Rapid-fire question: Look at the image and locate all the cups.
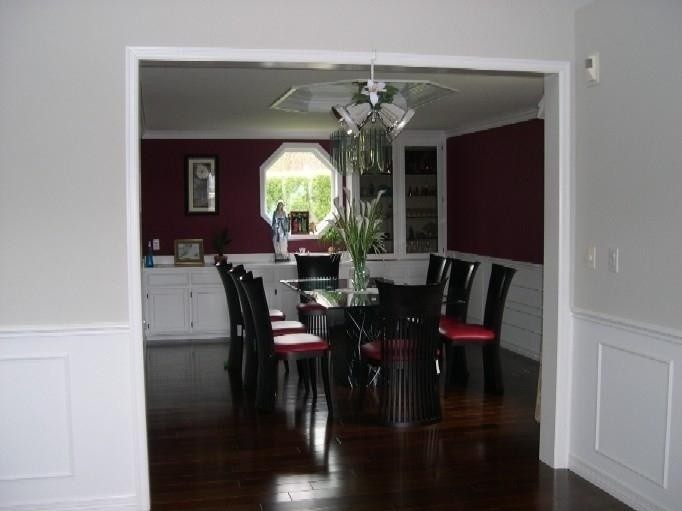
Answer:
[407,207,434,217]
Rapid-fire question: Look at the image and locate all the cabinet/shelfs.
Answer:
[142,264,190,340]
[335,129,450,261]
[191,262,230,340]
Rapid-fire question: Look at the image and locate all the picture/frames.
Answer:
[171,239,206,266]
[186,156,219,214]
[290,210,309,235]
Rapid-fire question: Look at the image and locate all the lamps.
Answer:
[329,48,416,174]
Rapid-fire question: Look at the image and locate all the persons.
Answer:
[291,217,307,232]
[271,202,290,262]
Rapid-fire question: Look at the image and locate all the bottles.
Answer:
[146,241,154,266]
[408,184,436,197]
[407,227,432,252]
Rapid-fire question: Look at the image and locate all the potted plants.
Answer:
[213,224,232,265]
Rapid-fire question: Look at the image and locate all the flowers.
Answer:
[325,189,392,292]
[351,79,400,112]
